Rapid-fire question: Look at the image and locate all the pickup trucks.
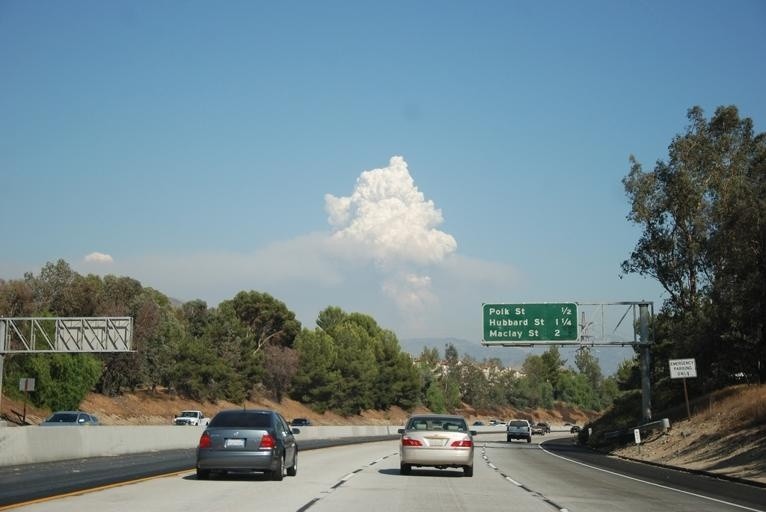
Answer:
[175,410,209,426]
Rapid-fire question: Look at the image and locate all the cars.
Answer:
[195,409,300,481]
[397,414,473,476]
[531,423,550,435]
[571,426,580,433]
[292,418,308,426]
[507,419,531,442]
[474,419,496,425]
[39,411,98,425]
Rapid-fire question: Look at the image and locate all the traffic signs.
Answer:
[482,301,581,344]
